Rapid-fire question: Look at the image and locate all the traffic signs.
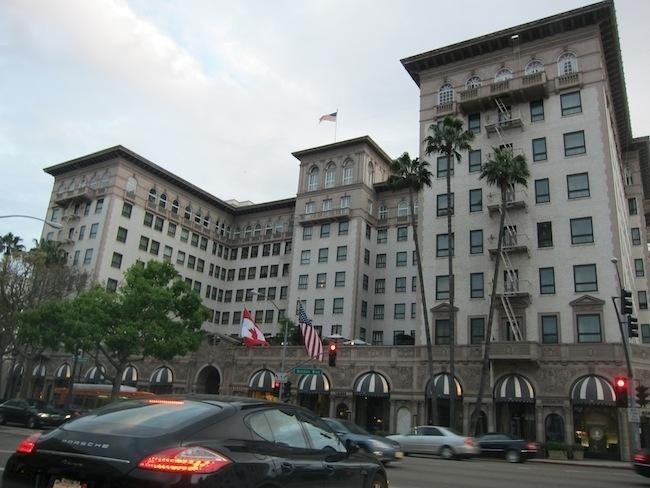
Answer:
[294,369,321,374]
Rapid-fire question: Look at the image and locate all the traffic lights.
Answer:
[274,381,280,390]
[615,377,629,409]
[621,288,633,316]
[635,386,649,407]
[328,343,337,367]
[629,318,638,337]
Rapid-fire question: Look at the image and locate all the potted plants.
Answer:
[543,441,587,461]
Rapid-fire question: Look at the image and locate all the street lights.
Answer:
[1,214,62,230]
[250,288,289,402]
[610,258,633,378]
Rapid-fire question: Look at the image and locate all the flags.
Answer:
[319,112,336,122]
[241,307,271,347]
[298,303,324,362]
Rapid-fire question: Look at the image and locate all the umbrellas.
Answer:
[324,331,347,340]
[343,336,372,346]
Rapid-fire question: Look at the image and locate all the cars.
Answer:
[475,432,539,463]
[387,425,482,461]
